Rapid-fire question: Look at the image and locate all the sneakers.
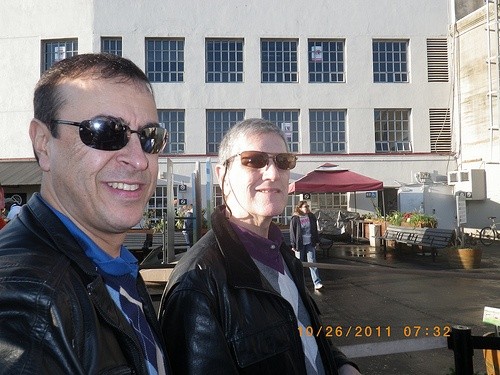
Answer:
[314,283,323,289]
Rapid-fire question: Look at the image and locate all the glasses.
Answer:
[0,208,6,214]
[222,151,298,170]
[301,205,310,208]
[53,115,169,154]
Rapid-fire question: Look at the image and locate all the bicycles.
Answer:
[480,217,500,246]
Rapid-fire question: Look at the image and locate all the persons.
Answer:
[156,118,363,374]
[0,52,167,375]
[0,185,23,230]
[179,204,195,252]
[290,200,325,290]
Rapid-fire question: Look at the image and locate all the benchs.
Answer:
[379,225,454,262]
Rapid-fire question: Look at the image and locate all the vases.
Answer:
[458,248,482,269]
